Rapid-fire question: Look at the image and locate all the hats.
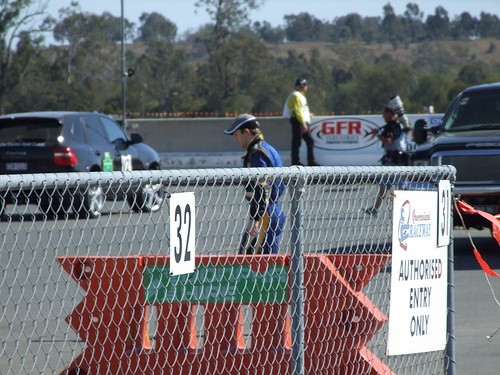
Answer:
[224,114,260,135]
[296,79,308,88]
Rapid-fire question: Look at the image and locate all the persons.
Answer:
[224,113,286,255]
[283,77,322,166]
[363,107,408,218]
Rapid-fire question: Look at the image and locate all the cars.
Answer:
[407,80,500,230]
[1,111,164,220]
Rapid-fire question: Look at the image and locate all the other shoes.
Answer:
[363,206,378,218]
[309,162,321,166]
[291,162,304,166]
